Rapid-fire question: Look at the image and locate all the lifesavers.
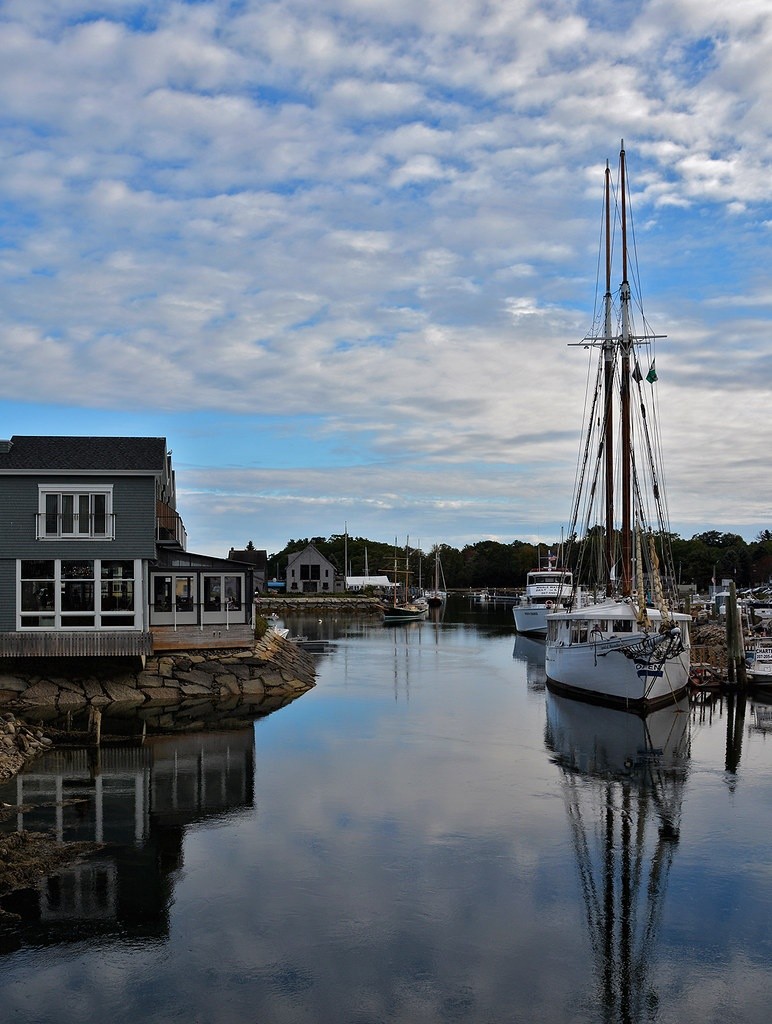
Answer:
[546,600,553,606]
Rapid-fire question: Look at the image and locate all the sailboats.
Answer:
[512,137,772,717]
[381,532,446,625]
